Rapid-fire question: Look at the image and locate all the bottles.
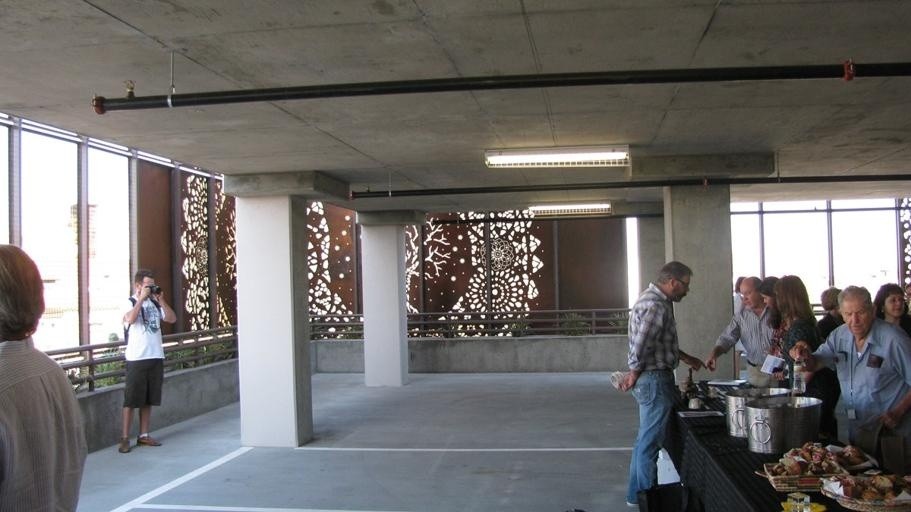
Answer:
[793,355,807,393]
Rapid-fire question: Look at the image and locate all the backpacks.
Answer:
[123,296,145,345]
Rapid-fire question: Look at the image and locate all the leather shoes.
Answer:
[137,434,162,447]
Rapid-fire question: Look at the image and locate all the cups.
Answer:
[689,398,701,409]
[611,372,625,389]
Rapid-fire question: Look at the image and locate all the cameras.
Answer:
[144,285,161,295]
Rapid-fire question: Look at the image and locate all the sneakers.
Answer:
[626,501,639,509]
[119,437,130,453]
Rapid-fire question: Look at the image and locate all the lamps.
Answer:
[527,198,612,217]
[484,143,632,168]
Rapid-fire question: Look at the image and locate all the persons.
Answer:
[705,274,911,476]
[0,243,89,512]
[118,269,177,452]
[618,260,707,506]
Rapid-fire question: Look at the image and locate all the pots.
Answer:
[724,387,790,438]
[743,396,824,455]
